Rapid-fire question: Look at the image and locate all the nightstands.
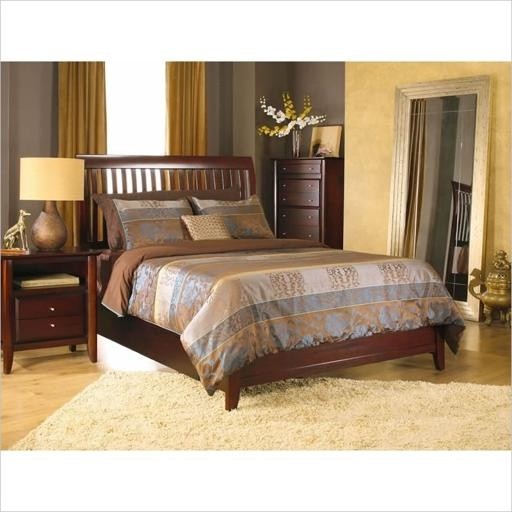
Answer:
[0,247,104,375]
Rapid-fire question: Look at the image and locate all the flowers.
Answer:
[256,88,328,138]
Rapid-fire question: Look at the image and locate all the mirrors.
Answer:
[384,71,497,325]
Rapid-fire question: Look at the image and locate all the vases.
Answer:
[291,128,305,158]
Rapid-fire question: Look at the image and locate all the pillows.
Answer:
[109,194,194,251]
[91,185,243,252]
[180,209,235,241]
[185,190,277,240]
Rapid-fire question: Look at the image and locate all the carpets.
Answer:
[8,365,512,451]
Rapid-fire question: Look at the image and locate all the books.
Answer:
[14,272,81,290]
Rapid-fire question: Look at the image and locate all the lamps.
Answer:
[17,156,87,253]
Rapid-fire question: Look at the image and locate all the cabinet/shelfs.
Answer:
[270,155,345,250]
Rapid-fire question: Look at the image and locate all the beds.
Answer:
[72,153,473,416]
[442,178,472,301]
